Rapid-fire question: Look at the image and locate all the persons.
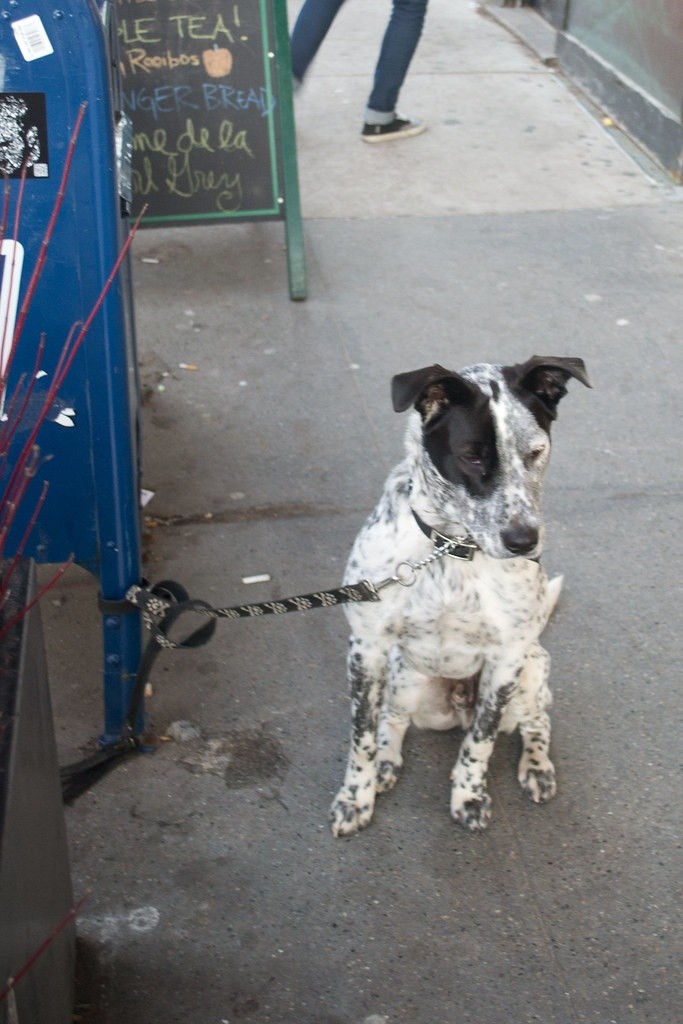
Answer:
[291,0,429,143]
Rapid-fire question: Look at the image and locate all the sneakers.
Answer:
[359,113,425,142]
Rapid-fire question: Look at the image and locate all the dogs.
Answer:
[327,353,594,839]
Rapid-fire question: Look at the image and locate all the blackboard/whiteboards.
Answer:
[107,0,286,221]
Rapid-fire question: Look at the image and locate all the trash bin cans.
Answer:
[0,0,184,794]
